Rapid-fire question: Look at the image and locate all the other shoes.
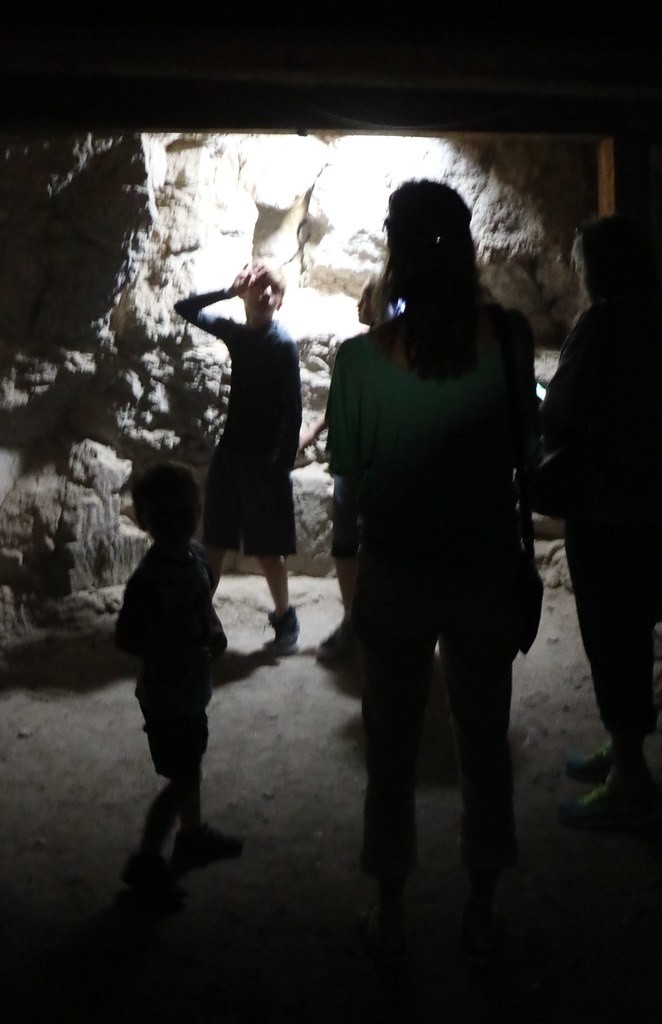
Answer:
[464,908,507,963]
[359,910,407,961]
[121,855,189,903]
[316,628,354,663]
[172,822,244,861]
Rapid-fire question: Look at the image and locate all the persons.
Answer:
[106,456,242,898]
[176,270,302,647]
[296,180,662,988]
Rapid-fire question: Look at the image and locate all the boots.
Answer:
[266,606,300,653]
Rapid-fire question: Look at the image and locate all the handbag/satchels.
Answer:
[516,549,544,655]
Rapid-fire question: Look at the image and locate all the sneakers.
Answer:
[565,743,613,780]
[560,768,658,827]
